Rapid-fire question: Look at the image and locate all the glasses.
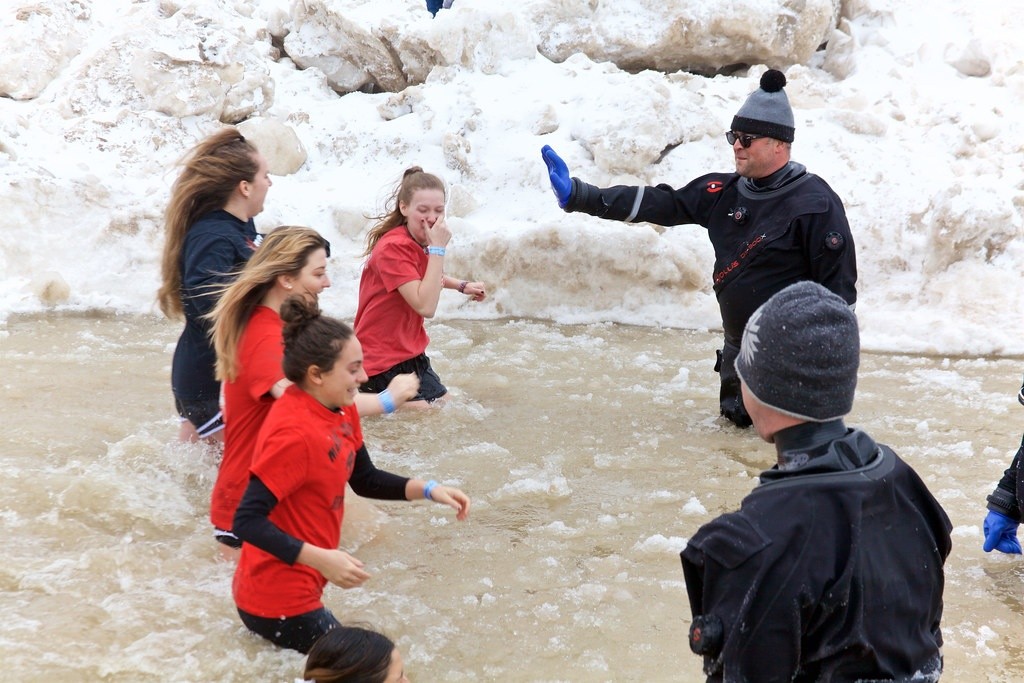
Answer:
[726,130,769,148]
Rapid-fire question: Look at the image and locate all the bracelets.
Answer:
[427,246,446,257]
[422,478,439,501]
[458,281,469,292]
[377,388,396,413]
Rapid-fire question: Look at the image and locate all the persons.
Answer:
[302,626,412,683]
[160,124,274,453]
[349,160,487,412]
[674,280,956,682]
[229,292,472,667]
[979,384,1024,553]
[187,225,334,561]
[542,65,861,437]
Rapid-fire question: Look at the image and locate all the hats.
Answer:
[731,69,795,143]
[734,279,861,420]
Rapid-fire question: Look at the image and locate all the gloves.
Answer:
[542,144,572,207]
[983,510,1022,555]
